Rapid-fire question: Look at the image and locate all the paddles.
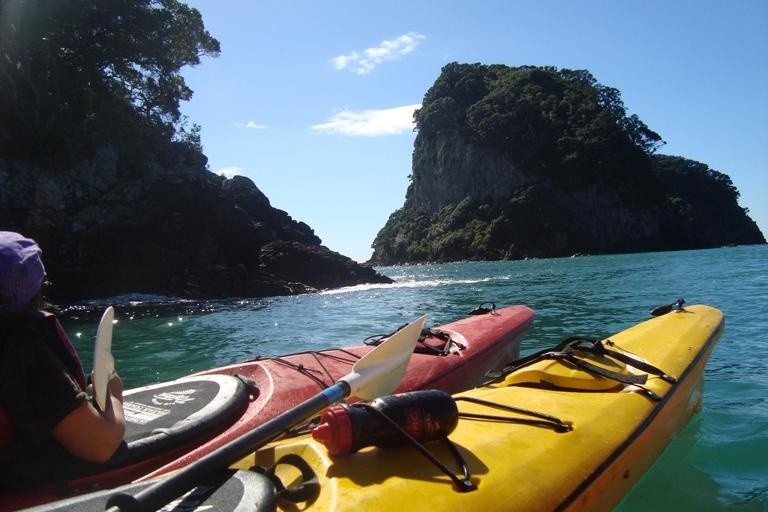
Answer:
[103,312,428,512]
[92,304,115,412]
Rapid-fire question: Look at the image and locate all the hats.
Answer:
[0,230,46,310]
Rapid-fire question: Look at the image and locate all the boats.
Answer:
[3,299,540,510]
[8,297,732,510]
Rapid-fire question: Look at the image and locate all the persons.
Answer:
[2,229,127,498]
